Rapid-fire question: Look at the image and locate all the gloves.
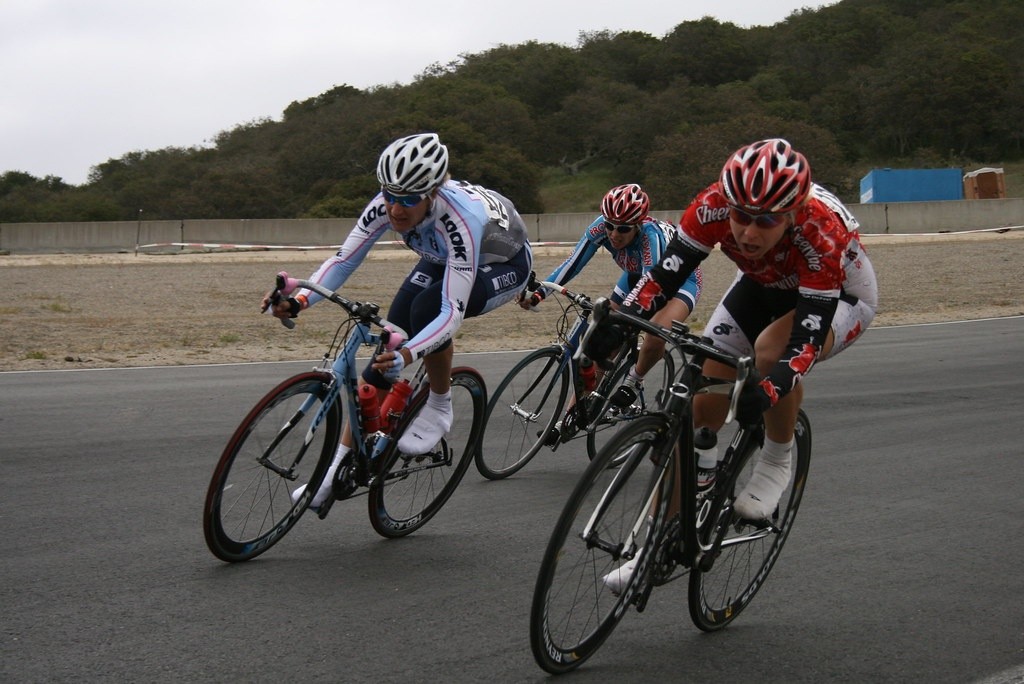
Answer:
[730,372,787,414]
[586,323,628,360]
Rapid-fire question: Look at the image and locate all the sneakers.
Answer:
[610,376,644,407]
[537,425,563,447]
[398,406,453,452]
[603,546,644,595]
[733,460,791,518]
[292,468,332,510]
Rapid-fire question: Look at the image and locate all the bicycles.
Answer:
[202,270,490,564]
[473,270,677,482]
[528,294,814,679]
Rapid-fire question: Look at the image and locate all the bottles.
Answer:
[380,379,414,434]
[579,356,598,392]
[359,384,381,432]
[695,425,718,493]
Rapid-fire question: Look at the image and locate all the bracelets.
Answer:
[534,293,543,303]
[299,295,309,309]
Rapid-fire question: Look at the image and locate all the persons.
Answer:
[263,131,532,510]
[514,182,701,446]
[600,139,879,596]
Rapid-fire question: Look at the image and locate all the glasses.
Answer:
[380,188,428,205]
[604,219,635,235]
[730,206,786,230]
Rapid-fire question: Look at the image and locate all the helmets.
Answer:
[721,137,810,211]
[376,133,448,194]
[602,183,650,224]
